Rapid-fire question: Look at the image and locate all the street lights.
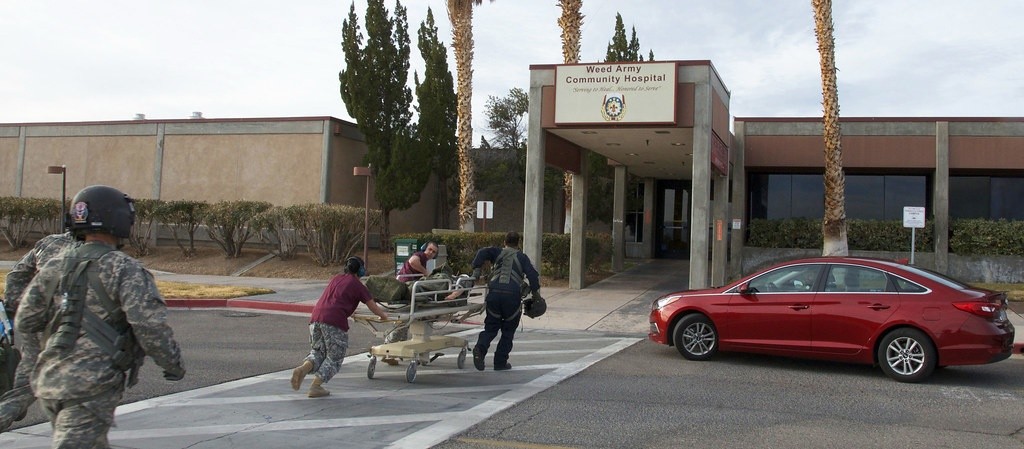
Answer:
[45,165,67,233]
[352,162,372,278]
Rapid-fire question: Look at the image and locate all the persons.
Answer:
[469,231,541,371]
[0,184,188,449]
[290,256,387,397]
[382,240,439,366]
[402,270,475,307]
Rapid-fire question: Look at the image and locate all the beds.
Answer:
[350,264,490,384]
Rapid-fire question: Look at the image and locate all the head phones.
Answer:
[343,257,365,277]
[421,240,439,259]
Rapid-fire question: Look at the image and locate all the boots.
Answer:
[291,361,313,391]
[307,374,330,398]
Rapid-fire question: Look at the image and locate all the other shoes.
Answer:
[474,348,485,371]
[493,363,511,370]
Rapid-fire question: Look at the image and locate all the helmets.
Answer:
[70,185,136,239]
[524,297,547,318]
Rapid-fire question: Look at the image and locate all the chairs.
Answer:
[843,272,860,292]
[824,272,840,292]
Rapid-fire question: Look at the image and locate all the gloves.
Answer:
[162,360,184,382]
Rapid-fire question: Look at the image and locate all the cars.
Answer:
[647,255,1017,385]
